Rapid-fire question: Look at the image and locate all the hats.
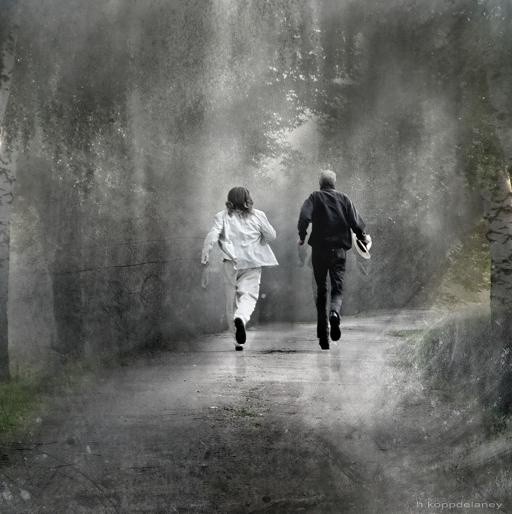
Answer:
[352,233,372,260]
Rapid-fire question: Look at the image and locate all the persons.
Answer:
[296,169,368,349]
[199,184,278,351]
[297,226,330,310]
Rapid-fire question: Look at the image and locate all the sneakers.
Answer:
[330,311,341,341]
[234,318,246,345]
